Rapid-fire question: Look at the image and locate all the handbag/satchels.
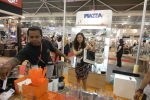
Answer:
[83,49,96,64]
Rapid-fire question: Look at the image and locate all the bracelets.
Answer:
[136,88,143,93]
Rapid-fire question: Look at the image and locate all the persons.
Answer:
[49,31,71,61]
[72,33,96,90]
[134,64,150,100]
[0,25,68,82]
[115,38,124,67]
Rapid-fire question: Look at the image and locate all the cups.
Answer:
[14,61,65,100]
